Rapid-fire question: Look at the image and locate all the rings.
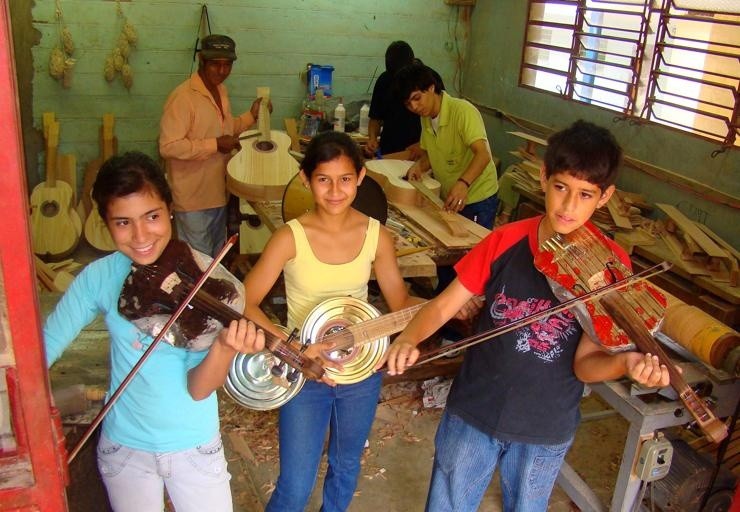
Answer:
[458,199,463,206]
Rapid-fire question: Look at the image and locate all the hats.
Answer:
[199,35,237,60]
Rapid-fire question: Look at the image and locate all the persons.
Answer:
[41,151,266,512]
[392,65,501,232]
[240,129,485,512]
[158,34,274,259]
[371,120,684,512]
[368,40,426,154]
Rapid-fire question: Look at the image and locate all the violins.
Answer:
[117,239,325,383]
[533,221,729,444]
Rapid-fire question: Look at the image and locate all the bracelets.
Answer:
[457,178,472,188]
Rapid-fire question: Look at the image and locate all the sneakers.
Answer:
[439,338,460,358]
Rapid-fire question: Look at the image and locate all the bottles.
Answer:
[333,103,346,133]
[359,101,370,137]
